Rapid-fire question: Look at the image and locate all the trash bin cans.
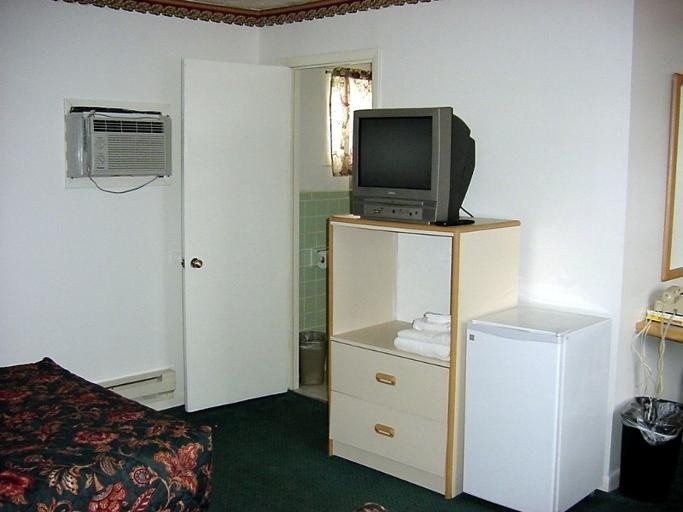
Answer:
[619,397,683,502]
[299,330,327,386]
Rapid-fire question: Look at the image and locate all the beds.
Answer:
[0,358,217,511]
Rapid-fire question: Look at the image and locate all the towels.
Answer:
[392,310,451,365]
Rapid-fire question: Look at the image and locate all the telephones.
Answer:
[654,284,683,319]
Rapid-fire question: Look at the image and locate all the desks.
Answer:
[634,319,682,344]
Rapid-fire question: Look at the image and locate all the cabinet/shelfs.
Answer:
[325,212,522,501]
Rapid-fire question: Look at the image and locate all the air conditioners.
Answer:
[63,107,174,182]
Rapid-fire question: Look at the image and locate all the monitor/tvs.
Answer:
[352,107,475,223]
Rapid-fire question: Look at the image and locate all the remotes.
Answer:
[435,219,475,226]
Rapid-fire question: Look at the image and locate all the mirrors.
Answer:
[660,72,682,282]
